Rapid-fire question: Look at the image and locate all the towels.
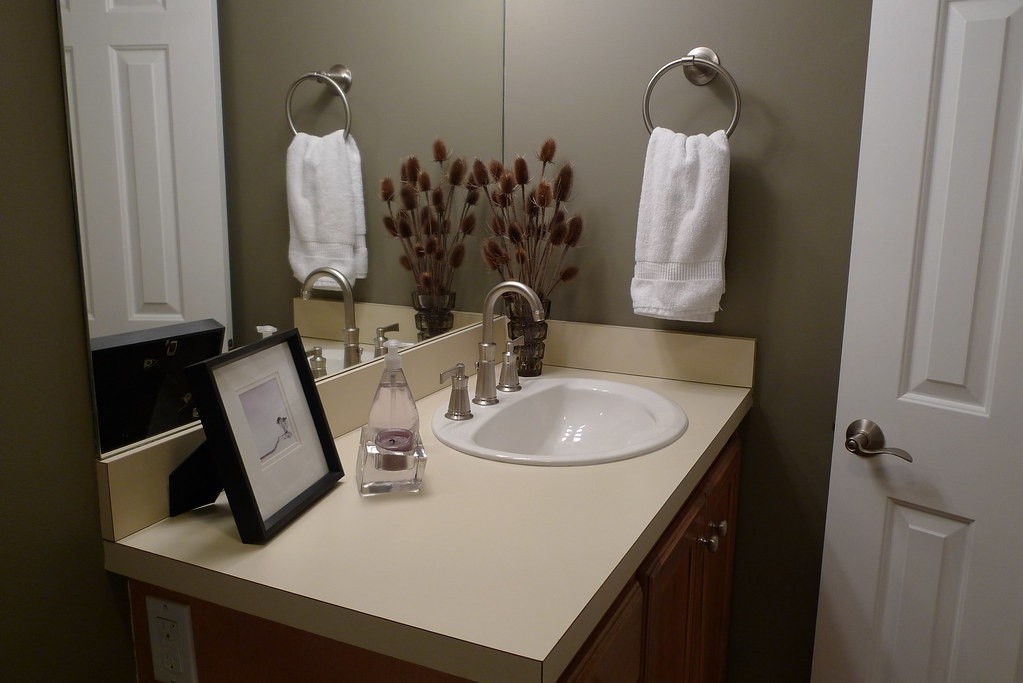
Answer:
[282,129,368,291]
[630,125,733,322]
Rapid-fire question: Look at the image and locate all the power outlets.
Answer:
[145,596,198,683]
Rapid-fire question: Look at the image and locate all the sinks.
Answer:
[430,377,691,467]
[301,346,375,375]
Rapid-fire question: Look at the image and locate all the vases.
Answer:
[411,291,457,344]
[504,300,551,378]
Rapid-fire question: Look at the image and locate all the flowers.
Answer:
[378,140,479,336]
[472,138,584,322]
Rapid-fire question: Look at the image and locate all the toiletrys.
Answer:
[254,325,278,339]
[369,337,419,430]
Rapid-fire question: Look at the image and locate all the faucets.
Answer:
[472,281,546,406]
[300,267,363,369]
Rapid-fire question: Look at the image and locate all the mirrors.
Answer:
[56,0,505,460]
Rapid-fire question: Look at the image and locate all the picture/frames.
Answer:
[184,327,344,545]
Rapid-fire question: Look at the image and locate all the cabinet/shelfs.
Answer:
[560,583,643,683]
[643,437,745,683]
